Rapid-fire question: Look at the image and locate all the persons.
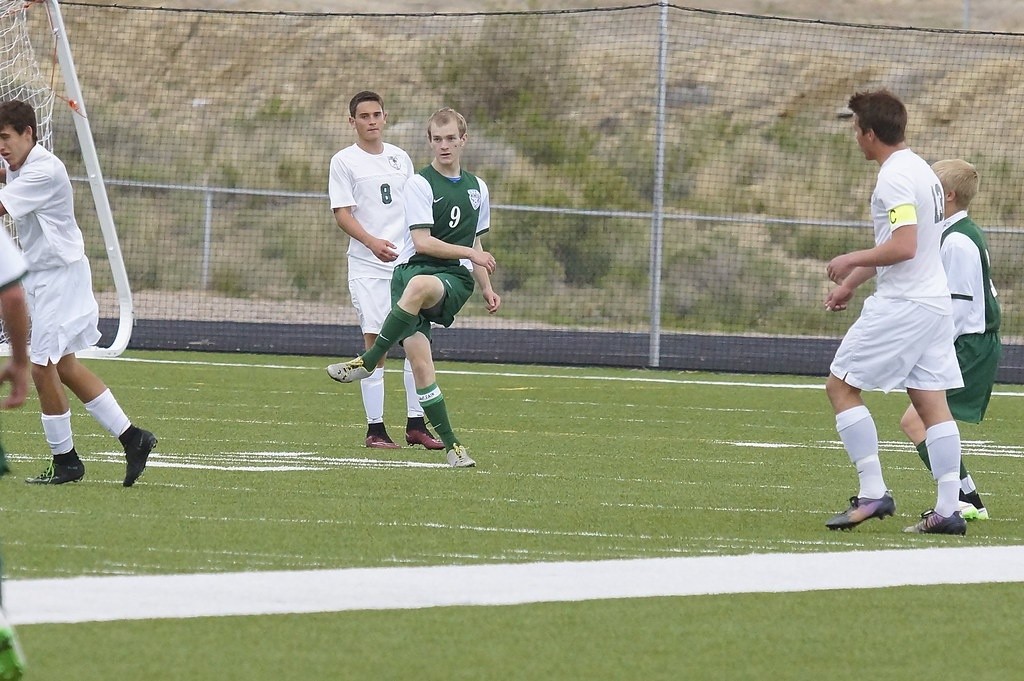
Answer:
[898,160,1001,520]
[328,90,446,451]
[0,102,158,487]
[1,226,30,407]
[824,90,969,535]
[325,106,502,468]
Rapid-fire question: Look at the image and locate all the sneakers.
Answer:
[26,448,85,485]
[825,489,897,532]
[117,425,157,487]
[921,500,989,520]
[365,423,401,448]
[406,417,445,450]
[326,355,378,383]
[445,443,477,468]
[902,507,968,537]
